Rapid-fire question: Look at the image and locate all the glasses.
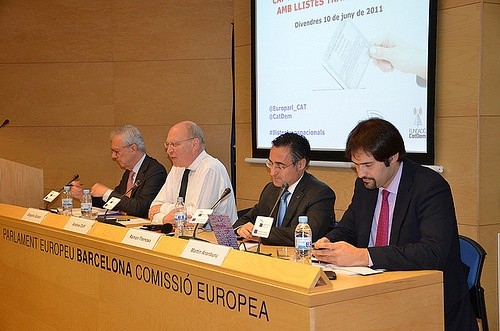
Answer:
[164,138,195,148]
[265,159,301,171]
[111,143,137,157]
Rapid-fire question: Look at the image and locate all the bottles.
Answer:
[173,197,187,236]
[79,189,92,219]
[61,186,73,216]
[295,216,312,263]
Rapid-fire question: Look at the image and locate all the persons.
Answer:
[148,120,238,231]
[309,118,479,331]
[231,133,336,246]
[69,125,167,215]
[369,37,426,79]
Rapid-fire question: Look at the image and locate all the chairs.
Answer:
[459,235,489,331]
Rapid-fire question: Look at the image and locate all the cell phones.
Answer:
[323,271,336,280]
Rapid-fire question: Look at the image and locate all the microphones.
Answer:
[178,188,231,243]
[97,185,137,227]
[39,174,79,214]
[247,182,289,255]
[0,119,10,127]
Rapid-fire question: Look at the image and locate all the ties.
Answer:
[177,169,191,206]
[375,189,390,246]
[126,172,136,198]
[276,192,291,227]
